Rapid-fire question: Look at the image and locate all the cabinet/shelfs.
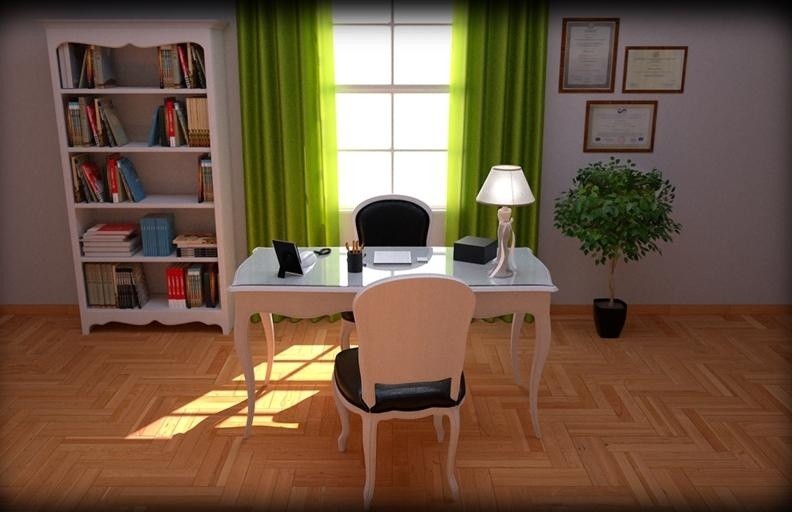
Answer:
[37,15,250,337]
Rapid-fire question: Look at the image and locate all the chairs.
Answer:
[334,274,478,511]
[340,197,432,350]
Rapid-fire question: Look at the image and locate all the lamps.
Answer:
[474,164,537,280]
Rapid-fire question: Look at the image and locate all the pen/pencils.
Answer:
[345,241,365,256]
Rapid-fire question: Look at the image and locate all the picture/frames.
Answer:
[557,16,690,154]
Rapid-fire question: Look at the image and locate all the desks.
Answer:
[226,245,559,439]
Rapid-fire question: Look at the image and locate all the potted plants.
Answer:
[552,155,683,341]
